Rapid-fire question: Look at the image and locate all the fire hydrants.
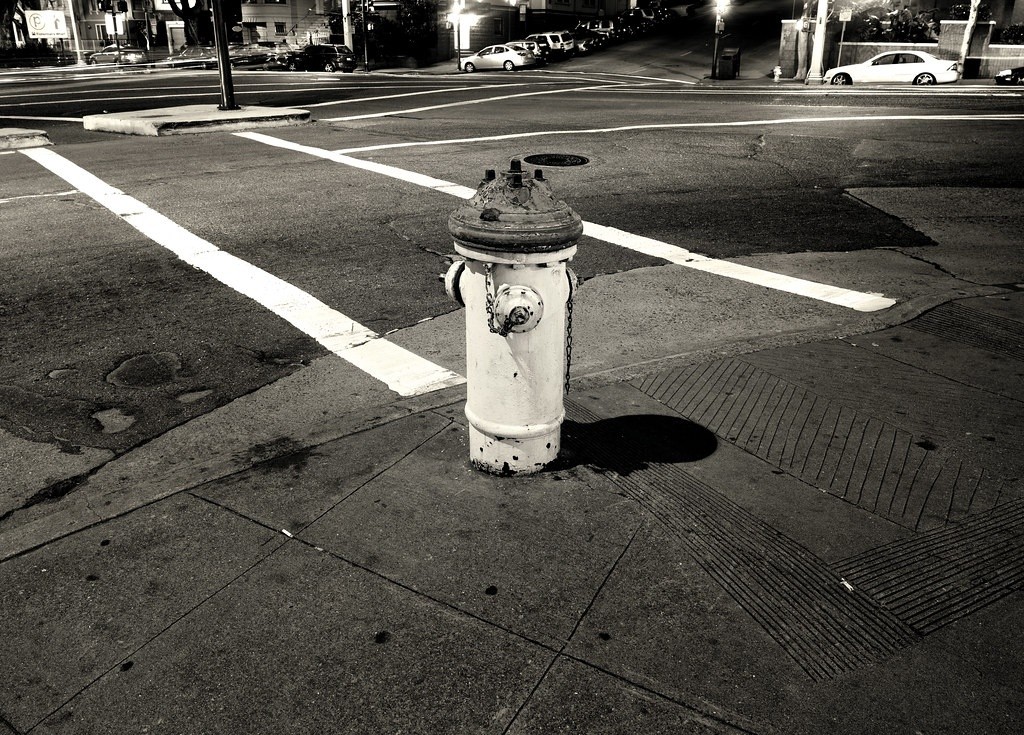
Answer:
[772,65,782,82]
[438,156,583,479]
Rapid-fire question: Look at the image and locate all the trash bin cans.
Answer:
[719,48,741,79]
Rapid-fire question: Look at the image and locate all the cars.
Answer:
[501,1,676,68]
[994,66,1024,85]
[460,43,537,73]
[88,43,149,69]
[822,48,961,86]
[166,40,359,73]
